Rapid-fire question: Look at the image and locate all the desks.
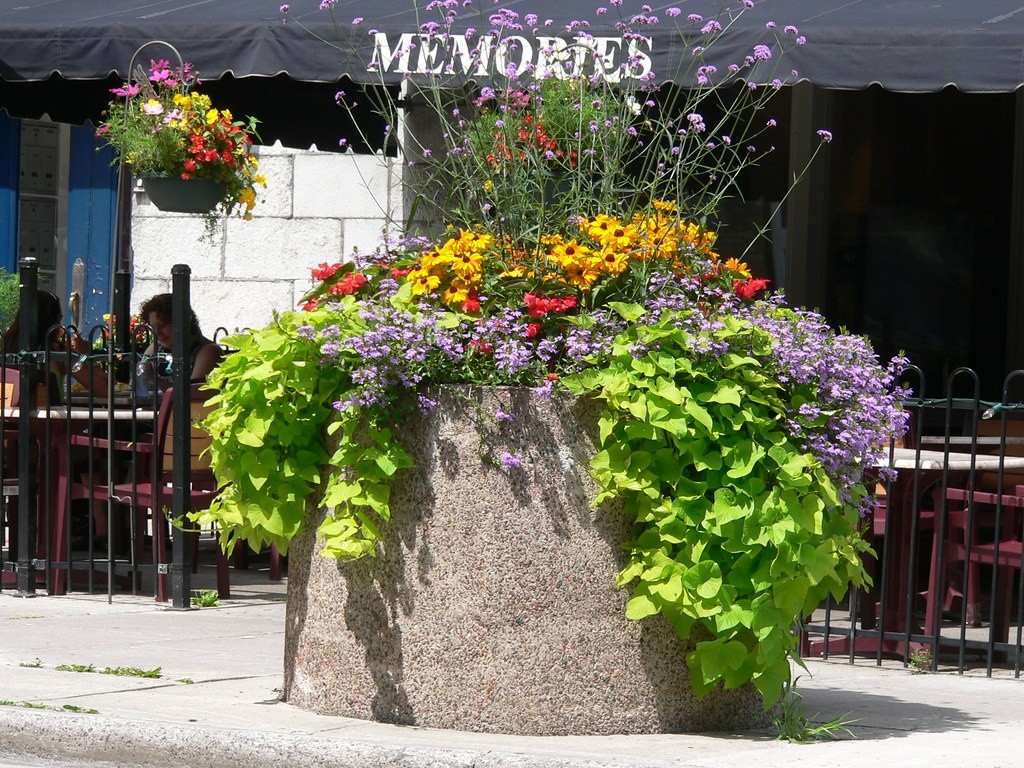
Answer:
[1,405,159,594]
[809,444,1024,670]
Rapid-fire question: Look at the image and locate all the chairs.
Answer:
[925,483,1024,663]
[0,366,20,407]
[48,381,230,601]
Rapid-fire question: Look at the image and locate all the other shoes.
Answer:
[71,533,108,553]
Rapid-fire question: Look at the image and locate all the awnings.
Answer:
[0,0,1024,158]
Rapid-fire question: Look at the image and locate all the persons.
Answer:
[0,289,109,555]
[130,294,224,395]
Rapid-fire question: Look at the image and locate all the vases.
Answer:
[140,173,227,213]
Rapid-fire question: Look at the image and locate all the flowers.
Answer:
[102,312,147,343]
[93,56,268,221]
